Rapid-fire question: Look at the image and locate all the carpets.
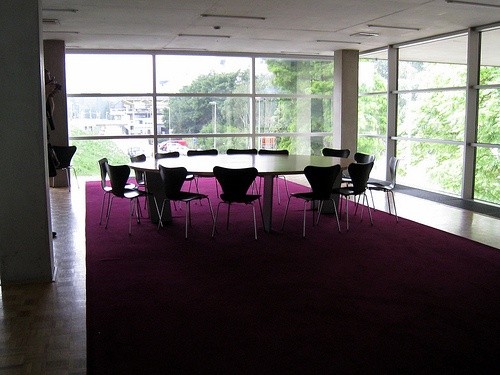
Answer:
[85,175,500,375]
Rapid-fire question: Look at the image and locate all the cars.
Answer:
[158,140,200,154]
[69,119,153,162]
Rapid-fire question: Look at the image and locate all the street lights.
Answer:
[156,106,174,159]
[209,101,217,149]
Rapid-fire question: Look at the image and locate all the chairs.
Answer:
[98,147,399,240]
[50,145,80,192]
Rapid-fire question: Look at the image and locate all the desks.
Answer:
[128,154,358,232]
